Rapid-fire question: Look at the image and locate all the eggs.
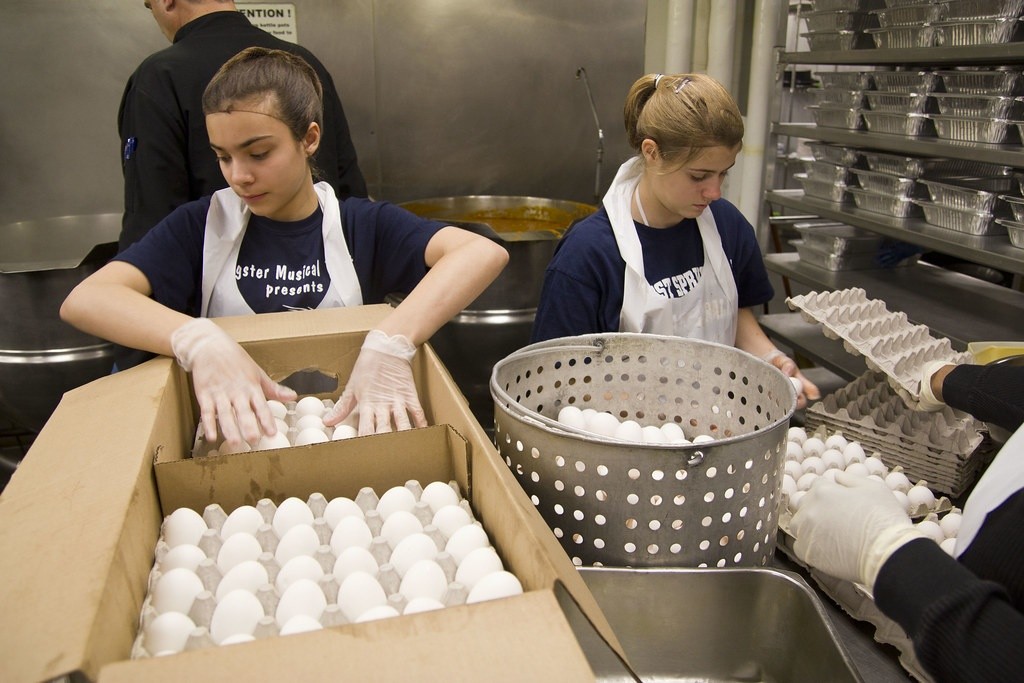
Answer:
[143,481,523,655]
[219,396,358,453]
[780,427,961,556]
[556,405,716,443]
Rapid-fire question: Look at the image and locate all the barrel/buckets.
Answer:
[384,195,600,431]
[0,215,128,487]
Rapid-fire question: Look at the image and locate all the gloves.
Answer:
[790,471,937,598]
[899,359,956,413]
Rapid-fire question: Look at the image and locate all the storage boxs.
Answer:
[0,303,641,683]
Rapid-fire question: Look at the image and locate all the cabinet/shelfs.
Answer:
[759,0,1024,418]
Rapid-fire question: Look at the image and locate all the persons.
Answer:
[532,73,823,408]
[788,352,1024,683]
[75,0,367,268]
[57,46,510,451]
